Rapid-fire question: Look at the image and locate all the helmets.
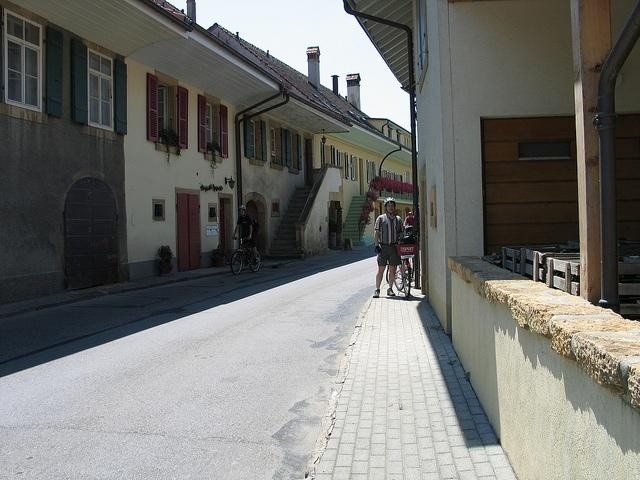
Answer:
[238,205,246,211]
[384,197,396,207]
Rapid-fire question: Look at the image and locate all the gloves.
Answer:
[374,246,381,253]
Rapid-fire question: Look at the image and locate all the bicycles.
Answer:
[386,223,419,301]
[229,236,262,275]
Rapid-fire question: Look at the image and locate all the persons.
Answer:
[403,211,415,228]
[371,196,403,298]
[232,204,259,266]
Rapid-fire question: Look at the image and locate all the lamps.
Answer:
[225,176,235,189]
[322,128,326,144]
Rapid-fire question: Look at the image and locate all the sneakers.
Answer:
[253,256,259,265]
[373,289,379,298]
[387,288,395,296]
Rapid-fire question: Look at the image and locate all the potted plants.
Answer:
[156,246,173,274]
[211,247,225,267]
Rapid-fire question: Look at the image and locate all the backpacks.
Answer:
[247,215,259,231]
